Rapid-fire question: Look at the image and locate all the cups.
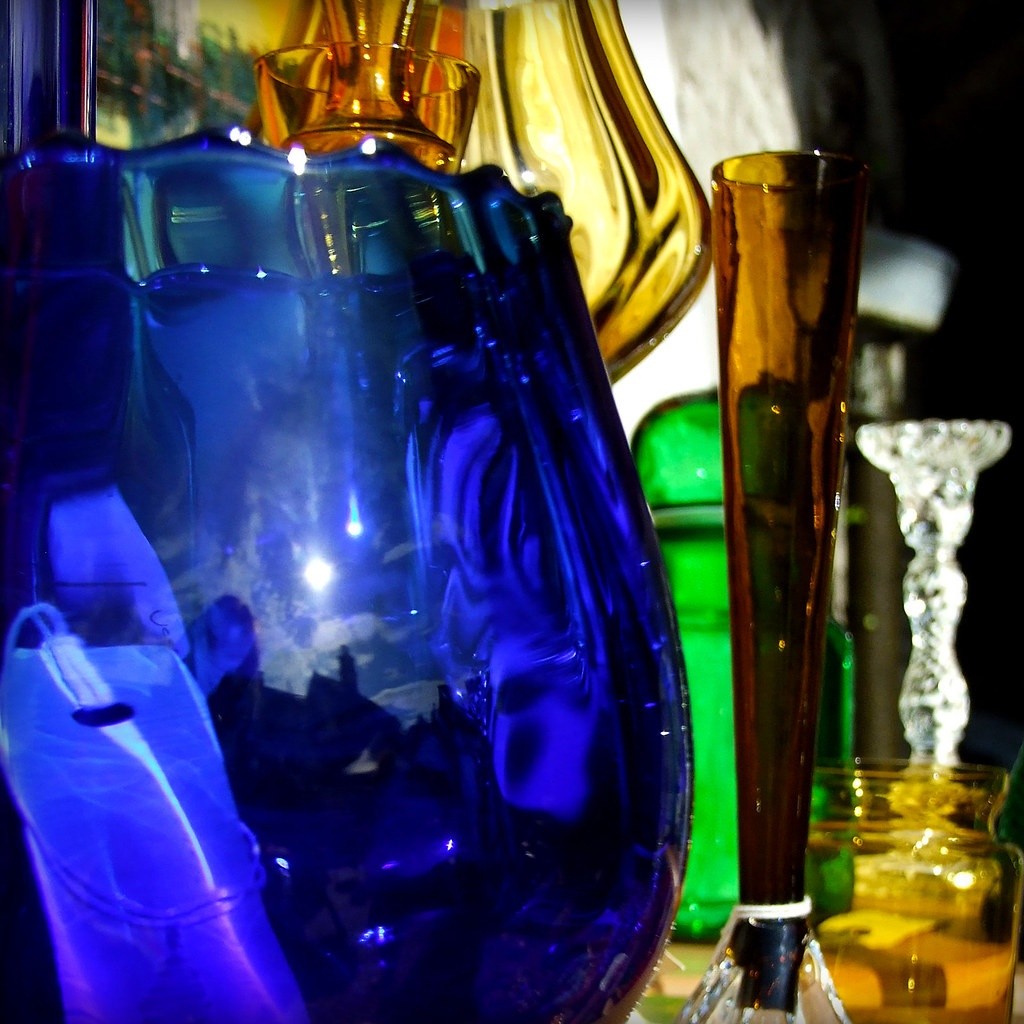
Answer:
[806,754,1024,1024]
[209,1,723,389]
[709,151,871,922]
[0,131,695,1023]
